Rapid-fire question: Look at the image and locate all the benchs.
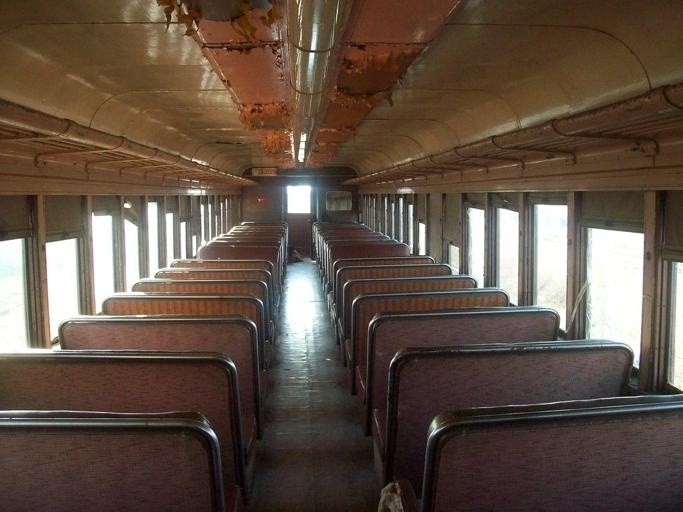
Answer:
[329,256,435,309]
[333,263,453,344]
[1,410,242,512]
[101,292,272,371]
[132,279,274,342]
[197,220,289,260]
[344,288,509,394]
[373,339,634,487]
[312,221,410,259]
[355,306,561,436]
[170,259,273,268]
[0,347,259,509]
[394,393,683,512]
[339,275,479,366]
[154,267,274,320]
[59,315,267,441]
